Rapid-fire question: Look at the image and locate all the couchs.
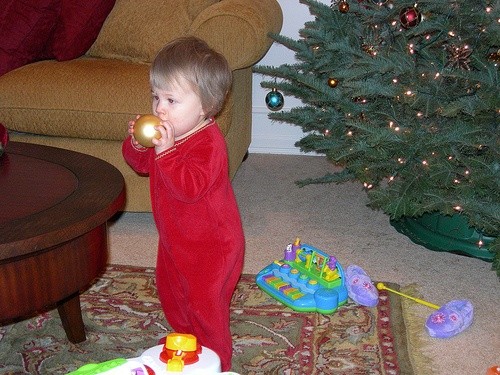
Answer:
[0,0,283,211]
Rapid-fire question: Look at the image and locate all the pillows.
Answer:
[0,0,116,77]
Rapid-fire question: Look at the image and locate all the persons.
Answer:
[120,38,246,372]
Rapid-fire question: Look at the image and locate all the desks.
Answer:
[0,142,127,343]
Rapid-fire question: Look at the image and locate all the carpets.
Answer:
[0,263,439,375]
